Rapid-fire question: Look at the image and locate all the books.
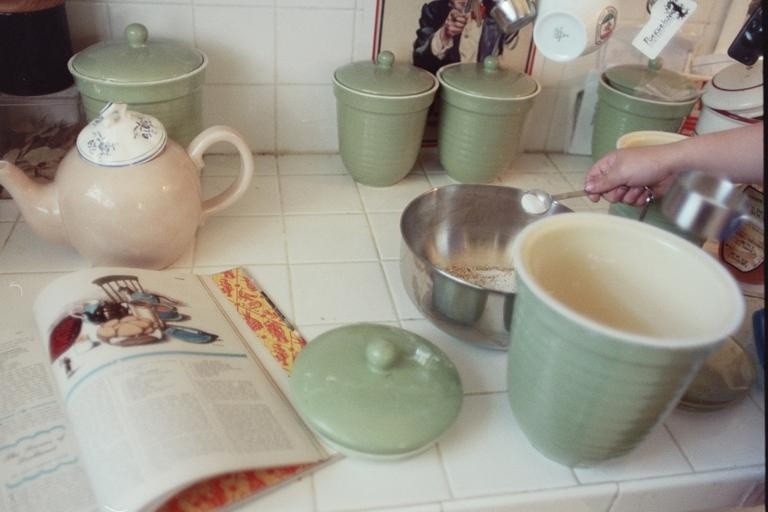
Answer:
[0,265,344,512]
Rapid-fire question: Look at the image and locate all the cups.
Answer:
[507,211,747,469]
[66,23,209,145]
[608,130,713,250]
[692,58,764,140]
[332,50,439,189]
[437,58,542,185]
[591,58,702,167]
[533,0,618,63]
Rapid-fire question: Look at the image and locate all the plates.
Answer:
[676,337,759,411]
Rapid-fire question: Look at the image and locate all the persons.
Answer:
[585,120,764,207]
[412,0,520,75]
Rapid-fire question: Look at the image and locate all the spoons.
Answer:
[520,181,656,215]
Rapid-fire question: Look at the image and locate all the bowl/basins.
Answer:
[490,0,535,35]
[400,184,573,352]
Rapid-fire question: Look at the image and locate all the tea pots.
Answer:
[83,301,129,324]
[0,102,253,271]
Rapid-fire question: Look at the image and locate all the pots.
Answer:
[661,170,764,242]
